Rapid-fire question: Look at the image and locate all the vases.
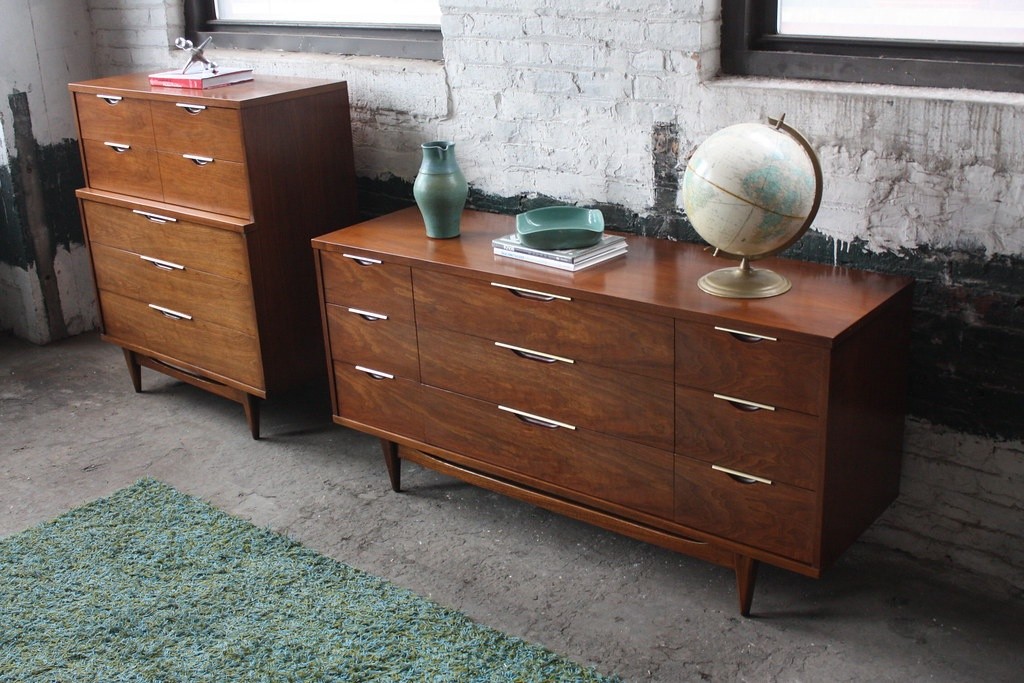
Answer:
[413,139,469,238]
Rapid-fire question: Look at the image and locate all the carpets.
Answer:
[0,476,625,683]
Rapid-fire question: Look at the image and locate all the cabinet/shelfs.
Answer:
[66,70,358,440]
[310,205,914,616]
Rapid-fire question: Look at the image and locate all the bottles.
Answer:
[413,141,468,238]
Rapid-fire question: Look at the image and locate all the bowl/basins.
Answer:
[517,206,605,250]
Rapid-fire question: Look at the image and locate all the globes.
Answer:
[681,113,824,299]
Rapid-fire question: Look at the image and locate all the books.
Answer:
[148,66,254,89]
[491,233,628,272]
